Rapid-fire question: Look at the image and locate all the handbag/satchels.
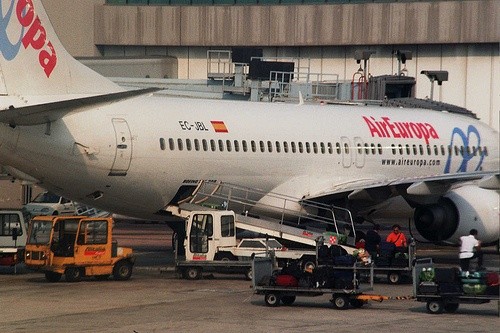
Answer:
[275,245,358,289]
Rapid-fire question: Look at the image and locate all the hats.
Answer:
[316,236,324,242]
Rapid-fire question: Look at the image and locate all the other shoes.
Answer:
[476,267,486,270]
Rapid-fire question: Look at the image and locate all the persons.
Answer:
[385,224,408,249]
[364,223,381,264]
[458,229,486,273]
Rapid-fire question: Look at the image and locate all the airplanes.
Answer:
[0,0,500,255]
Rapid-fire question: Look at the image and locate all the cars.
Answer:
[24,191,84,218]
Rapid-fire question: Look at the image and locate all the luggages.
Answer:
[417,266,500,296]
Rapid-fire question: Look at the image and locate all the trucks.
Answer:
[171,210,317,280]
